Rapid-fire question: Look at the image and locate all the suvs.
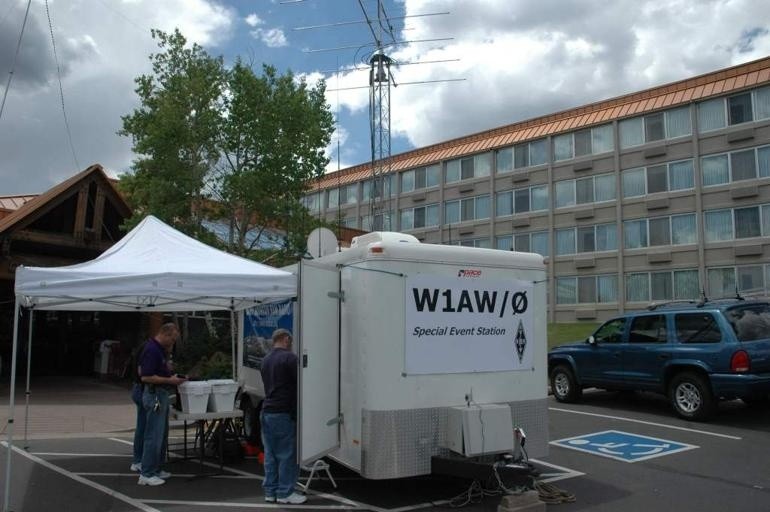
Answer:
[547,287,769,421]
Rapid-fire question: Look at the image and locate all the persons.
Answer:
[130,322,188,486]
[260,328,308,504]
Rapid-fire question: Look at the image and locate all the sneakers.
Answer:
[264,493,307,504]
[137,470,171,486]
[131,462,142,472]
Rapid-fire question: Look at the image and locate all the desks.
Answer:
[168,405,245,477]
